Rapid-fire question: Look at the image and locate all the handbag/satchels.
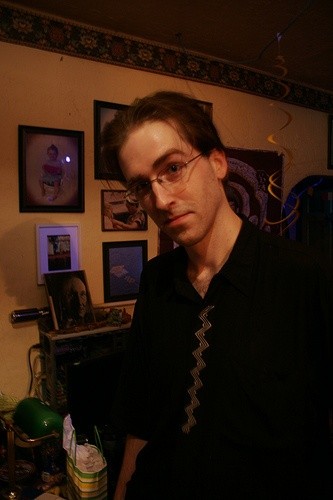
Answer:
[66,425,108,500]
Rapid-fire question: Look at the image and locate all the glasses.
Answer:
[124,146,217,203]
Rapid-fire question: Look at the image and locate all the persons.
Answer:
[103,201,118,229]
[98,89,333,500]
[58,276,95,331]
[38,143,66,202]
[111,190,146,230]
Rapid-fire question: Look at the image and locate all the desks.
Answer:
[38,303,144,410]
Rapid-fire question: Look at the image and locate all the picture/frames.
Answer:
[102,240,147,304]
[18,125,85,213]
[92,97,133,182]
[35,223,85,286]
[41,271,95,333]
[101,190,148,232]
[224,147,285,238]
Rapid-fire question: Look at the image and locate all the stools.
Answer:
[0,407,63,492]
